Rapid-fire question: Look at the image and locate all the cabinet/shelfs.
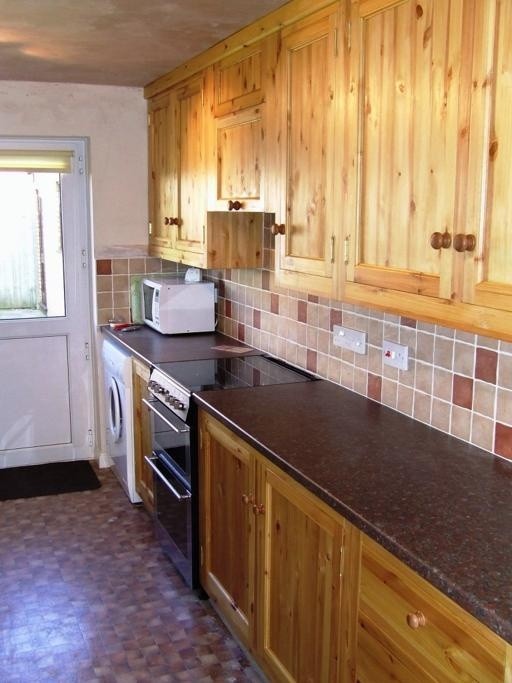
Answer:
[145,54,263,270]
[344,0,512,342]
[196,406,346,683]
[270,0,344,278]
[206,15,277,213]
[344,524,512,683]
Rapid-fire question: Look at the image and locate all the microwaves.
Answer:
[138,276,215,335]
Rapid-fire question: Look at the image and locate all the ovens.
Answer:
[140,393,199,591]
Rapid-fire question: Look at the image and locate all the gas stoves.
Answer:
[147,354,316,424]
